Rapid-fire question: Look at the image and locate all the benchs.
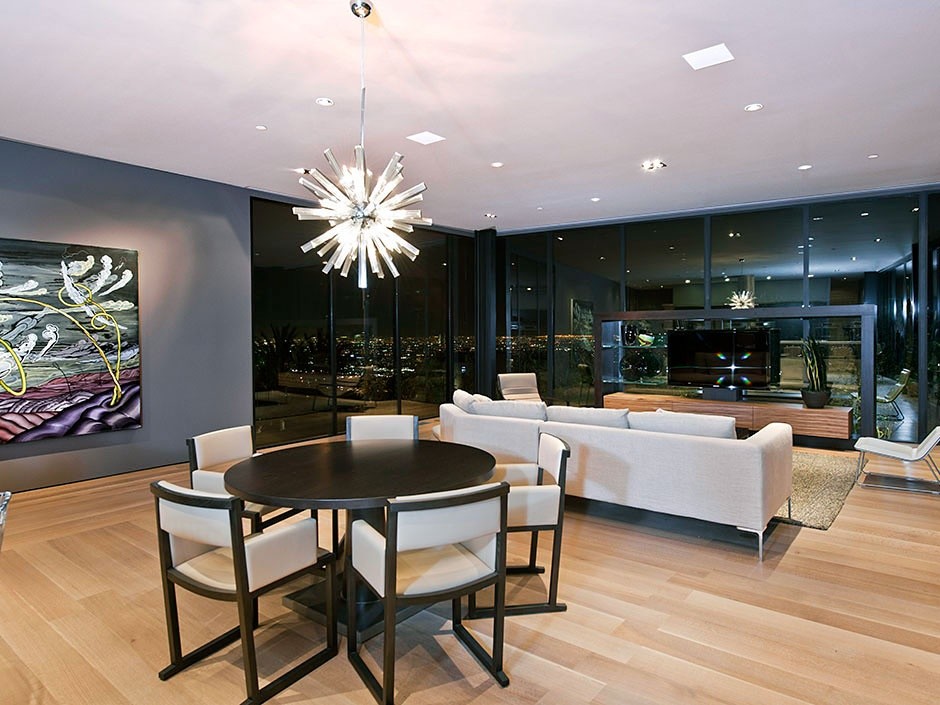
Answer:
[430,387,794,565]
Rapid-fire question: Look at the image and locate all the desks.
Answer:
[604,392,854,441]
[223,439,497,640]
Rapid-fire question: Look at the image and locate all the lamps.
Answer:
[293,0,433,288]
[724,258,758,310]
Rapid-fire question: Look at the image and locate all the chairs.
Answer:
[577,364,594,406]
[876,369,911,419]
[854,425,940,494]
[498,373,543,402]
[151,415,573,705]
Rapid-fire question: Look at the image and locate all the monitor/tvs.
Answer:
[665,329,774,388]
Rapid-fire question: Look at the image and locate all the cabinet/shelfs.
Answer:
[597,314,724,394]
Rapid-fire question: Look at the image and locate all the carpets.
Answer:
[777,451,869,530]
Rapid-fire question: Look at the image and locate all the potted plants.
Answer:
[802,336,831,409]
